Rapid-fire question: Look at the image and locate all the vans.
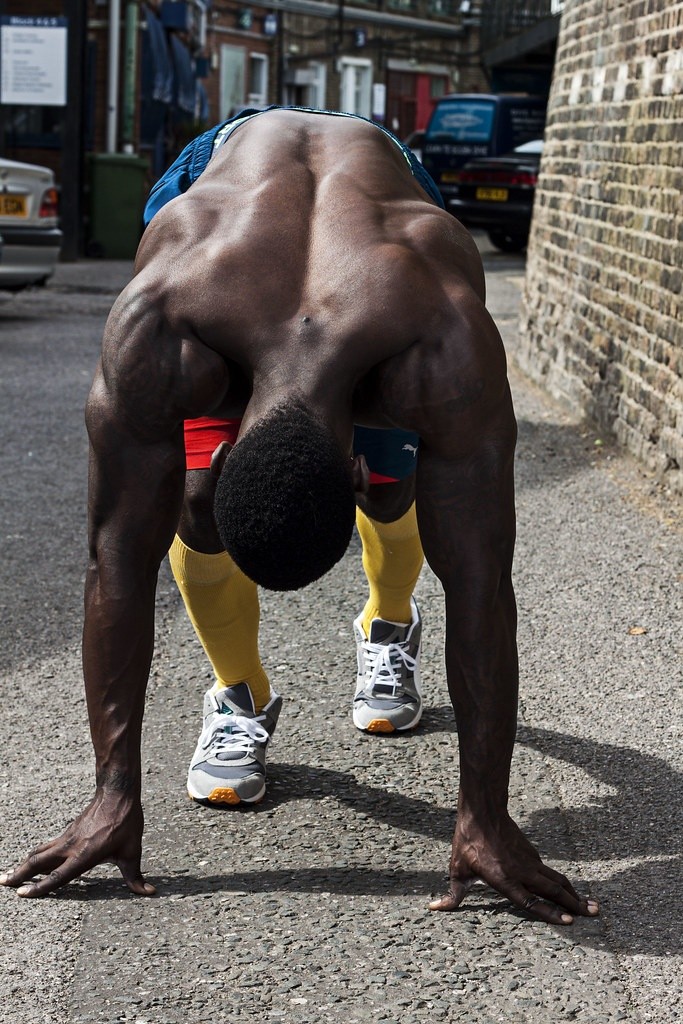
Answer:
[422,90,547,232]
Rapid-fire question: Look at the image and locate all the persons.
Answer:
[0,102,601,924]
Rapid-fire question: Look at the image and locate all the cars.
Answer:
[0,158,64,292]
[448,137,546,258]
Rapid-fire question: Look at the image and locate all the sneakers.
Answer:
[187,678,283,804]
[353,592,421,734]
